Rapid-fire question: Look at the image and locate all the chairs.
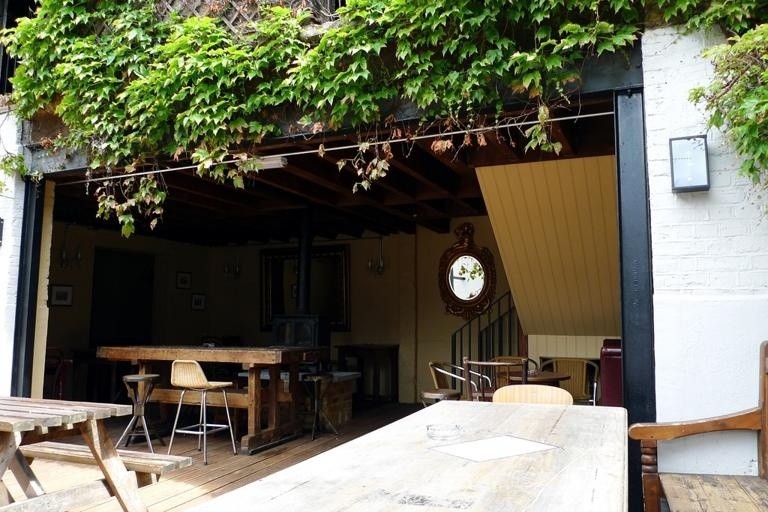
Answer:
[167,358,238,466]
[416,354,600,406]
[19,439,193,510]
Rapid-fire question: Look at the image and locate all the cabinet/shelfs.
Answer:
[600,338,622,406]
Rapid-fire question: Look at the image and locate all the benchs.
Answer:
[628,340,768,511]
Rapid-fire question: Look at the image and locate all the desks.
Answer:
[0,393,149,512]
[45,339,400,455]
[184,399,632,512]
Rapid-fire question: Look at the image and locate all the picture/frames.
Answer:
[175,270,193,290]
[48,285,75,308]
[191,292,206,310]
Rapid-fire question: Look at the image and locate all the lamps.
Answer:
[667,134,711,196]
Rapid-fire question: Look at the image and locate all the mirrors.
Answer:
[438,223,498,321]
[258,243,352,333]
